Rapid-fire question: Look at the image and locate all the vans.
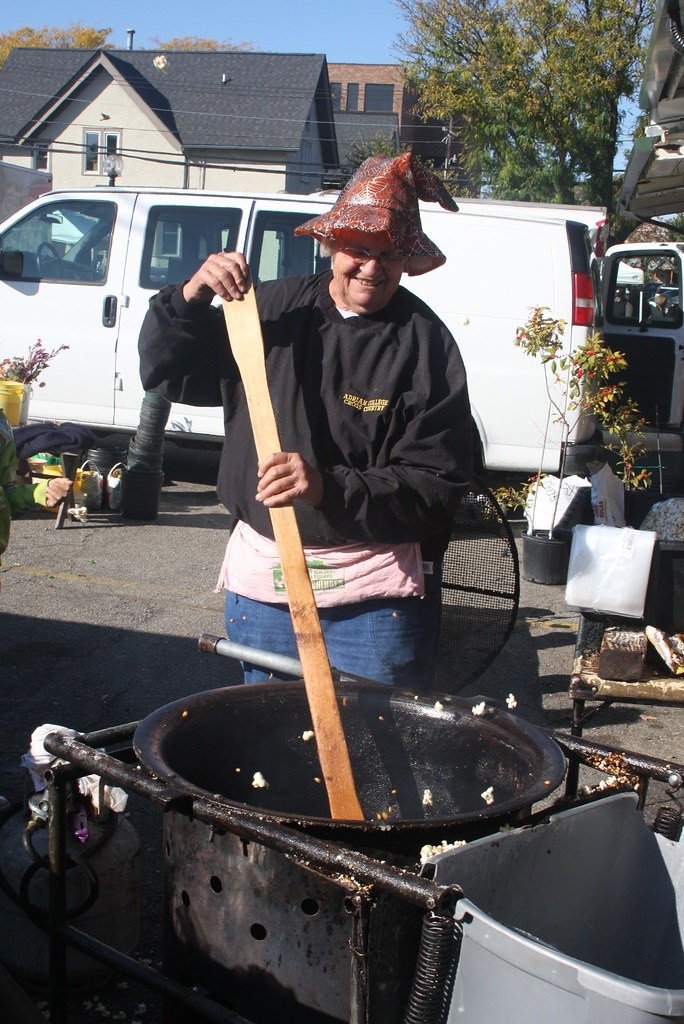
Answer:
[0,185,684,475]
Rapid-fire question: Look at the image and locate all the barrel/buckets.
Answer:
[121,468,164,520]
[0,381,24,427]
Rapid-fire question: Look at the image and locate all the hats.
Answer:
[294,151,459,276]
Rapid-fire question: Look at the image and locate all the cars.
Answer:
[612,261,680,314]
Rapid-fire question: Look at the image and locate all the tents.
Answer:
[615,261,645,323]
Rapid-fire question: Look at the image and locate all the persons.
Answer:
[139,150,477,692]
[0,408,74,810]
[649,295,668,319]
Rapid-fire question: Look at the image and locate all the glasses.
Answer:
[336,240,406,263]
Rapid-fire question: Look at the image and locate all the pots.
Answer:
[132,681,567,872]
